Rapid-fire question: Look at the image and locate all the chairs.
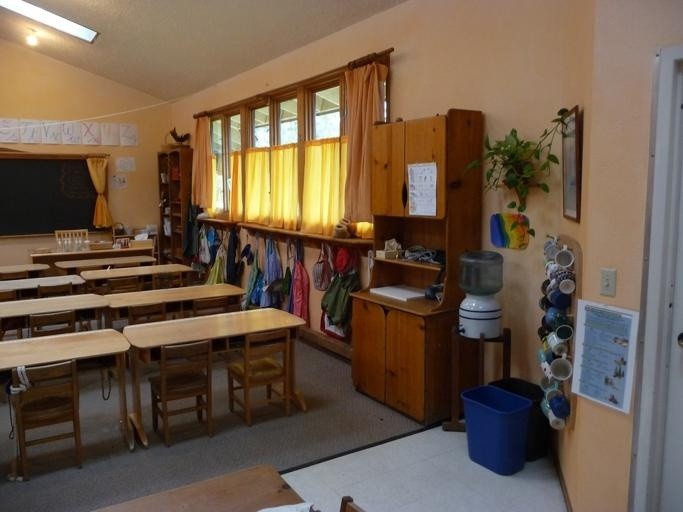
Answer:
[0,262,307,482]
[340,495,368,512]
[55,229,89,249]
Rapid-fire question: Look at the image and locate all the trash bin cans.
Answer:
[460,385,532,476]
[488,377,544,462]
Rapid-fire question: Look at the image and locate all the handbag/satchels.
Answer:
[313,243,331,291]
[199,224,211,264]
[283,240,291,295]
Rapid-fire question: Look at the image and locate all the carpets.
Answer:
[276,417,567,511]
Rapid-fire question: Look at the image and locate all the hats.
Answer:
[331,247,357,274]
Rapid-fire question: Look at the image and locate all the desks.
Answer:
[30,247,155,264]
[54,255,157,275]
[92,463,316,512]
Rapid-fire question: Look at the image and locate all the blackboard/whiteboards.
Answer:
[0,152,109,239]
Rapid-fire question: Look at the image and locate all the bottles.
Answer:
[58,237,87,252]
[163,217,171,236]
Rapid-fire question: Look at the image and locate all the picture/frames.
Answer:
[561,104,580,223]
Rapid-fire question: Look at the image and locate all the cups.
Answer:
[124,224,133,235]
[164,208,171,215]
[161,172,167,182]
[538,234,577,431]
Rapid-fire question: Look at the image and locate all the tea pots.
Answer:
[112,222,125,235]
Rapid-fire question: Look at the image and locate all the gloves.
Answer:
[332,225,350,239]
[339,220,353,234]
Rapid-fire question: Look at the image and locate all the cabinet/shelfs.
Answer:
[111,225,160,285]
[347,110,483,426]
[157,147,193,265]
